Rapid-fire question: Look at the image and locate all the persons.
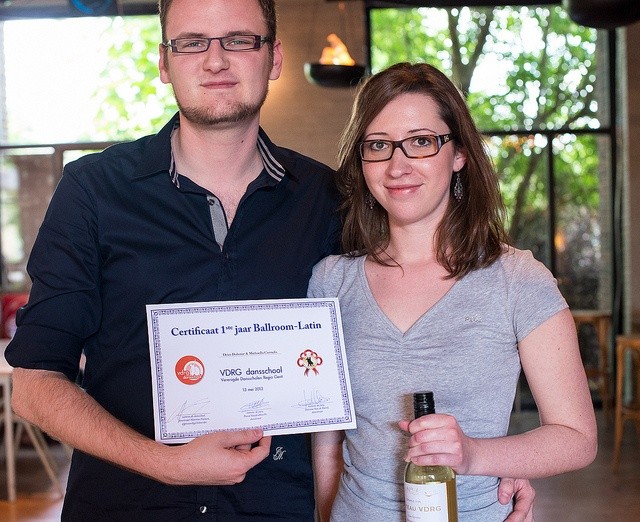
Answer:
[304,60,601,521]
[4,1,537,521]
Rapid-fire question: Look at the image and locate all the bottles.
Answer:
[404,391,458,522]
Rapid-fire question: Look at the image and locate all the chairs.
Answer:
[0,337,75,502]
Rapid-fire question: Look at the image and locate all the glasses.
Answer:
[164,34,273,54]
[360,131,458,163]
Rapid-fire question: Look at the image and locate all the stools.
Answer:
[610,336,640,474]
[570,309,612,448]
